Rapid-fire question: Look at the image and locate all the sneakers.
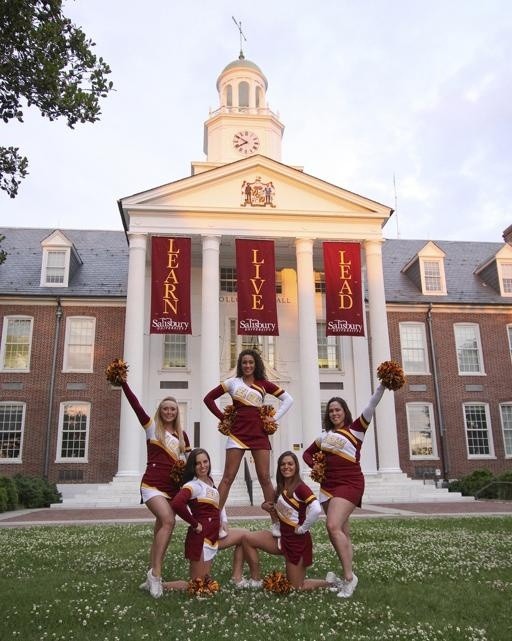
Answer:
[326,572,359,598]
[218,529,227,539]
[272,522,281,538]
[244,578,263,588]
[139,569,164,599]
[229,575,248,588]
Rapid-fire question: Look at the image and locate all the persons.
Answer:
[139,449,267,593]
[120,378,190,598]
[203,349,293,539]
[302,378,386,599]
[242,450,343,593]
